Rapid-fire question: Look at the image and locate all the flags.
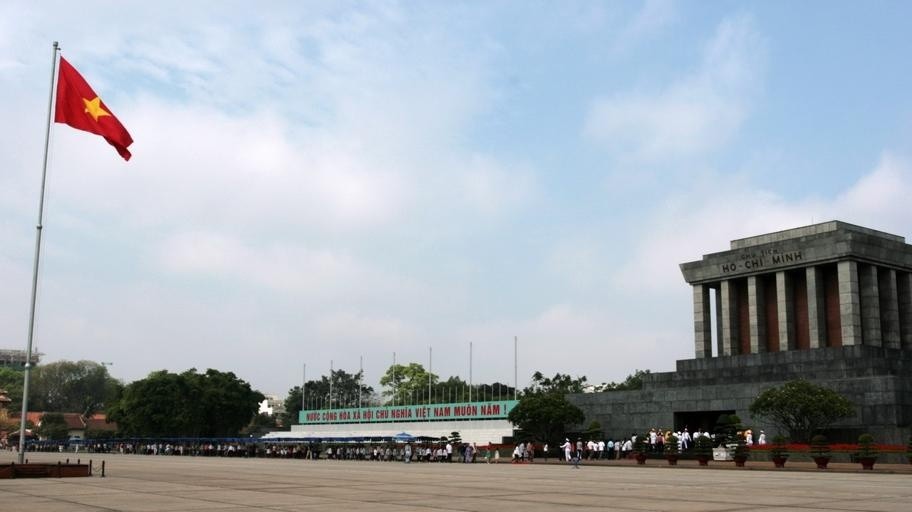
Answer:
[55,55,133,161]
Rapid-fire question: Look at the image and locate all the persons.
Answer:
[95,442,260,458]
[513,423,767,465]
[263,439,503,463]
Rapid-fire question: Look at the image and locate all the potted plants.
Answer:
[634,430,881,471]
